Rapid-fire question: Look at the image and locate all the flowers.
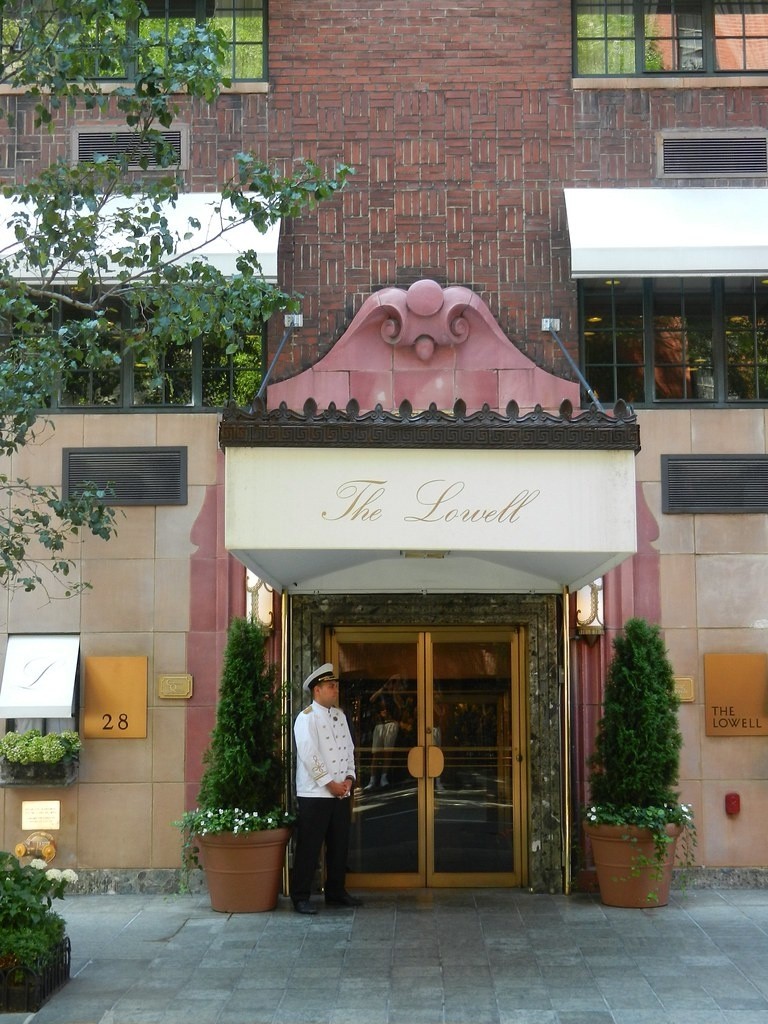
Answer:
[0,850,79,984]
[572,803,698,904]
[172,807,296,900]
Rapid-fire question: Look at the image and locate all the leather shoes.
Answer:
[294,900,317,914]
[325,894,363,905]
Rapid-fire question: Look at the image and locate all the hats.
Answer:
[303,663,339,691]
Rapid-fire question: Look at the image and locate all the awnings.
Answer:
[0,633,80,718]
[0,193,282,286]
[563,187,768,278]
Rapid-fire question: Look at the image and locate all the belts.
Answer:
[376,721,394,724]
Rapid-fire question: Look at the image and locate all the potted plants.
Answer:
[197,615,293,913]
[0,728,83,779]
[580,617,686,907]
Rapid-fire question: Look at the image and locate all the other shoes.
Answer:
[363,783,376,790]
[380,781,389,787]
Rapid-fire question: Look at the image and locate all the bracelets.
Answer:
[382,686,385,690]
[346,777,353,781]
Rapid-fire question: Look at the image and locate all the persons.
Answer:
[289,662,364,914]
[364,674,459,792]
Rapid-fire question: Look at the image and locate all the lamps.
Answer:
[573,577,606,647]
[246,567,275,639]
